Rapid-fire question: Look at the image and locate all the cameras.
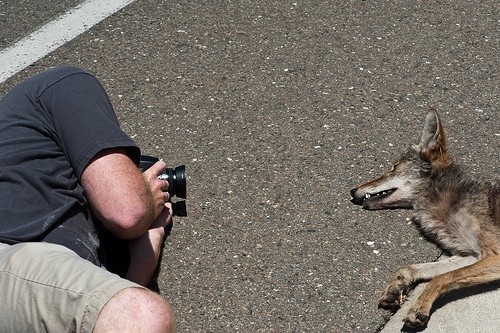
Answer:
[126,146,187,199]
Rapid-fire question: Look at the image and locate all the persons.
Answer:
[1,65,178,333]
